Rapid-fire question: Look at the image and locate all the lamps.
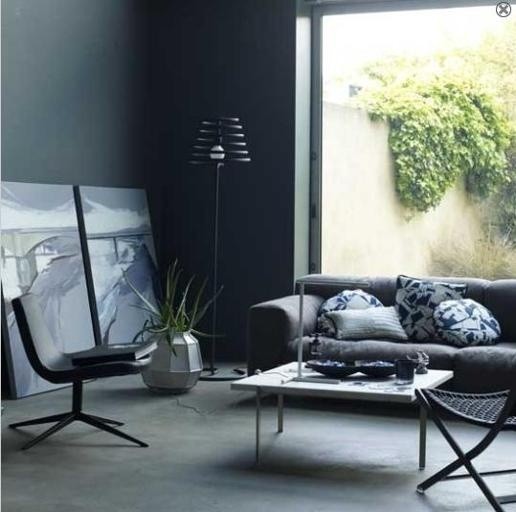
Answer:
[188,114,249,381]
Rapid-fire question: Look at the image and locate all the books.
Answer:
[69,340,160,366]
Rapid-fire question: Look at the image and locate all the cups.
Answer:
[396,360,415,384]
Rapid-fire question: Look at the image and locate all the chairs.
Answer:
[9,293,153,451]
[414,359,516,512]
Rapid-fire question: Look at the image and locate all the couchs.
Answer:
[246,273,516,421]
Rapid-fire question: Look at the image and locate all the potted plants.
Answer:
[120,254,231,397]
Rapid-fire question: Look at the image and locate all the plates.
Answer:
[307,358,394,378]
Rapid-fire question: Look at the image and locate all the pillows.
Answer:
[317,275,502,349]
